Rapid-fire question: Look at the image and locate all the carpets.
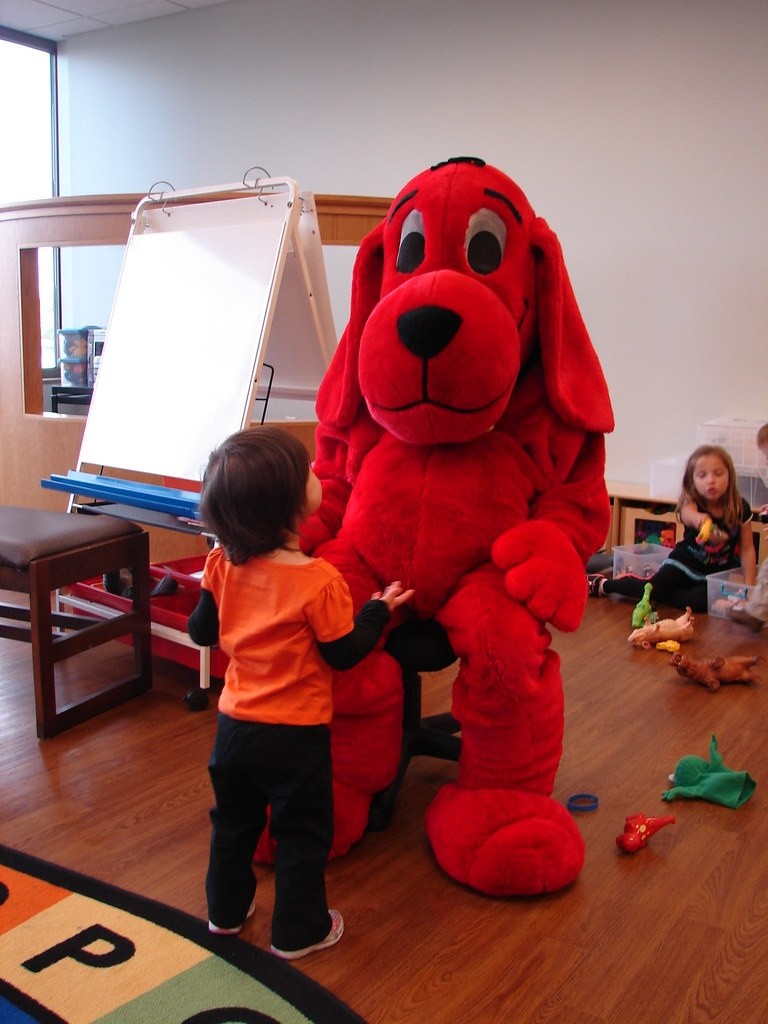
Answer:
[0,844,366,1024]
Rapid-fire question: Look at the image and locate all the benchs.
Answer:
[51,385,94,414]
[0,505,150,741]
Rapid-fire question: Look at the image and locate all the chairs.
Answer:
[367,613,464,834]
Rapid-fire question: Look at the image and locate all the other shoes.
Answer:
[270,910,343,959]
[581,573,605,596]
[208,898,255,933]
[728,601,763,630]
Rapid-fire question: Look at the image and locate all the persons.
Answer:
[188,427,416,959]
[724,423,768,632]
[586,446,756,614]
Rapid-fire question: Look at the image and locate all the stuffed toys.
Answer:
[253,157,614,901]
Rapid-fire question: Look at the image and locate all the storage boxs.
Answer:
[60,359,88,387]
[611,544,673,580]
[57,325,102,363]
[703,417,768,477]
[705,566,762,621]
[650,456,689,501]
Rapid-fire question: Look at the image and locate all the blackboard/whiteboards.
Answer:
[74,192,305,495]
